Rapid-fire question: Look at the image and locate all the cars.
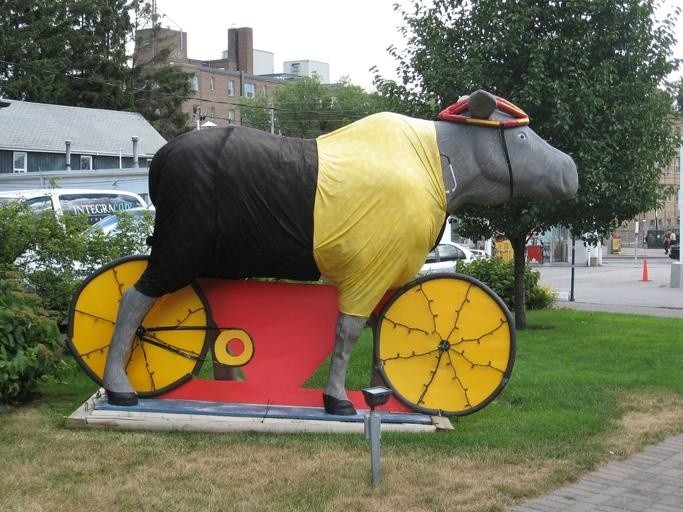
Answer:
[670,244,680,259]
[0,188,156,280]
[420,242,488,274]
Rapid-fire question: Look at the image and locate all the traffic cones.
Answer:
[638,259,652,281]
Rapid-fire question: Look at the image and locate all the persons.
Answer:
[662,230,680,254]
